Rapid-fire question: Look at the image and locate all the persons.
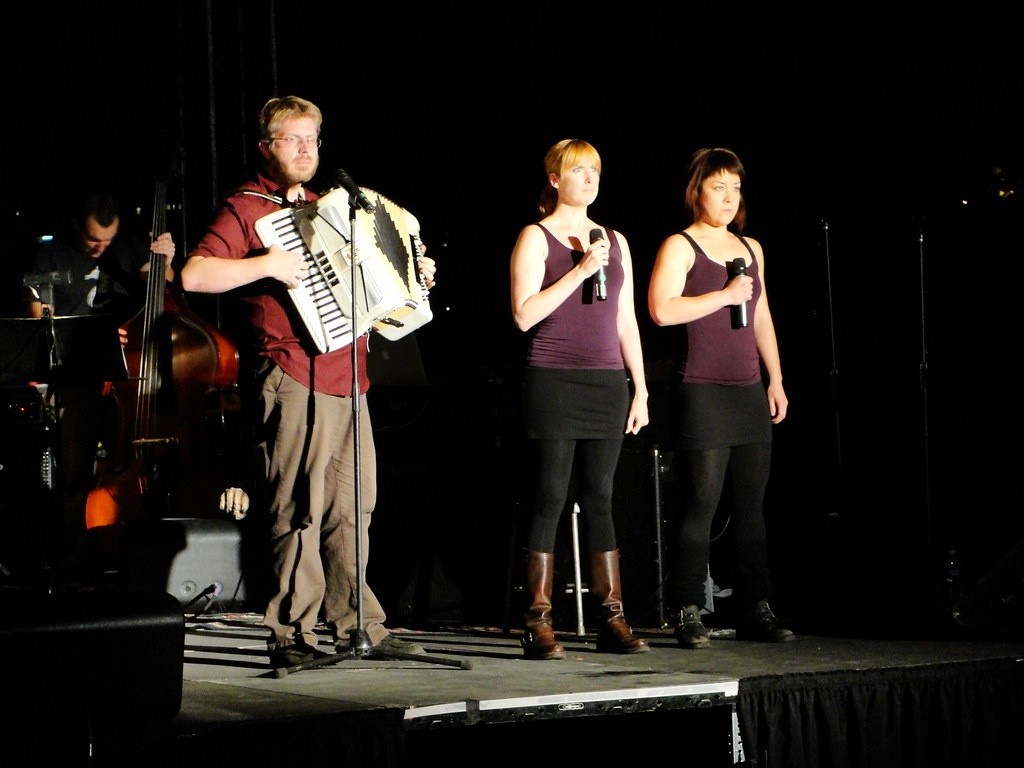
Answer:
[23,197,176,372]
[648,147,797,650]
[510,138,649,659]
[181,96,437,672]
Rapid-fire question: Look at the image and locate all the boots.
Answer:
[585,548,651,653]
[519,552,566,659]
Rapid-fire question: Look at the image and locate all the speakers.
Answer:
[0,515,244,754]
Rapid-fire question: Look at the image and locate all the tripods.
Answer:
[271,193,471,678]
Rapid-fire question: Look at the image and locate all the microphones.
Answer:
[732,258,748,328]
[589,229,608,302]
[336,169,375,214]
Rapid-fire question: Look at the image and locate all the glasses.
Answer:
[265,137,321,148]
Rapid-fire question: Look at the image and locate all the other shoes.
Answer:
[269,644,336,667]
[335,633,426,661]
[736,600,795,642]
[674,604,711,648]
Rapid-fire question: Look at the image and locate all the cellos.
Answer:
[79,142,242,578]
